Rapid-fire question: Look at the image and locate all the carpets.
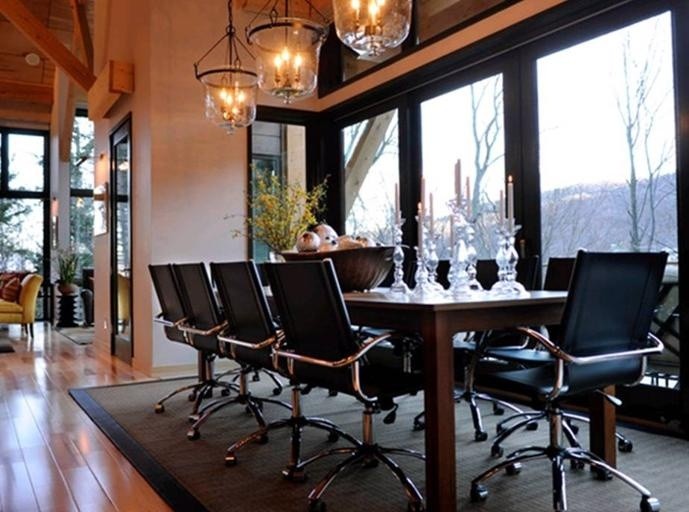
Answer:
[67,372,689,512]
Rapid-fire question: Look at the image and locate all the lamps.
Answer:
[331,1,412,56]
[192,1,258,135]
[243,1,328,104]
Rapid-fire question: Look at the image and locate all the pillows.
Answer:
[1,277,19,302]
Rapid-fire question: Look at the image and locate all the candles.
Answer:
[507,175,514,230]
[394,182,399,222]
[421,177,425,214]
[457,159,462,204]
[465,176,471,217]
[449,214,454,247]
[454,163,456,194]
[499,189,504,224]
[417,203,422,248]
[429,193,432,230]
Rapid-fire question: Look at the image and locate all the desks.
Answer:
[195,291,618,511]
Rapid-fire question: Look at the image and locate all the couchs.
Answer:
[0,271,43,339]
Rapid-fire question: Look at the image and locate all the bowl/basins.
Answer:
[280,243,409,293]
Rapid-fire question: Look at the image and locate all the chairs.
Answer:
[470,250,670,511]
[411,254,543,442]
[210,260,365,485]
[478,256,634,470]
[149,265,261,420]
[206,365,285,397]
[265,257,427,512]
[170,263,295,445]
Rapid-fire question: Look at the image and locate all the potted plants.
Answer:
[221,159,331,262]
[52,249,88,295]
